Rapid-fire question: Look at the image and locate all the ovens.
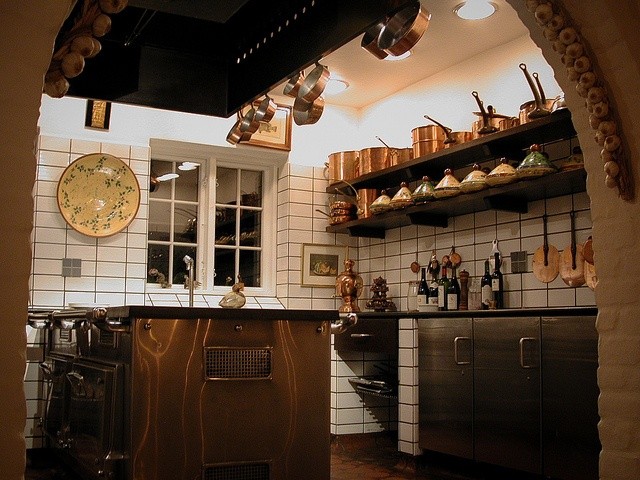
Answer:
[27,313,330,480]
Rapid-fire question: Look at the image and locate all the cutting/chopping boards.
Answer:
[532,214,560,282]
[558,210,586,288]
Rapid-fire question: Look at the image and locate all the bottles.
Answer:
[407,280,420,313]
[491,252,503,309]
[438,265,449,311]
[481,261,492,310]
[416,268,429,311]
[468,279,483,310]
[428,268,439,304]
[447,266,461,311]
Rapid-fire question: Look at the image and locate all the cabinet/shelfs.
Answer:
[182,211,260,255]
[417,318,470,463]
[541,314,597,478]
[471,314,538,474]
[325,108,587,239]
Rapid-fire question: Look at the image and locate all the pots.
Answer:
[283,71,306,98]
[360,19,389,60]
[519,62,555,126]
[293,96,324,126]
[377,1,432,56]
[297,61,330,103]
[240,102,260,134]
[424,115,472,149]
[254,93,277,122]
[240,133,251,141]
[472,90,519,139]
[226,110,245,145]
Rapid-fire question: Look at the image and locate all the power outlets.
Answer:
[510,251,528,273]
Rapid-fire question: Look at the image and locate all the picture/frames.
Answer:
[239,100,293,152]
[85,97,111,131]
[301,242,349,289]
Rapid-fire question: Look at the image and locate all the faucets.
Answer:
[182,253,198,305]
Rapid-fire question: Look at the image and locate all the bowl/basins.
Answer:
[328,201,357,225]
